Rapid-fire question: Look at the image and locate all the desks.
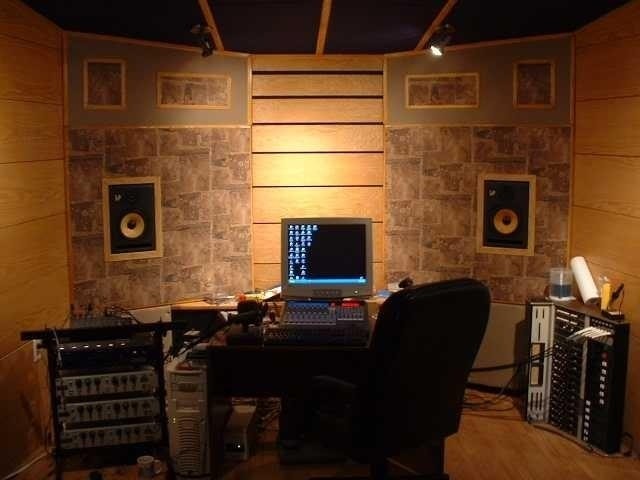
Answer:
[187,300,399,480]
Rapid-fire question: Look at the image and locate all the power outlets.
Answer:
[33,341,43,362]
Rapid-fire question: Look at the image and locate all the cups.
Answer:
[136,455,162,478]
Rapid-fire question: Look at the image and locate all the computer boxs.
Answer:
[163,349,211,479]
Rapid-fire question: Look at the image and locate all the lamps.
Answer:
[429,26,454,56]
[191,25,215,58]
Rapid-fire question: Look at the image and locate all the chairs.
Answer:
[317,277,492,478]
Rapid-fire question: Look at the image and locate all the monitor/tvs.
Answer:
[280,216,373,301]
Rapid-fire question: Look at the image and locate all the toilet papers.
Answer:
[570,256,600,303]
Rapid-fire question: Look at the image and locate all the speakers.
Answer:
[108,183,156,254]
[483,180,529,250]
